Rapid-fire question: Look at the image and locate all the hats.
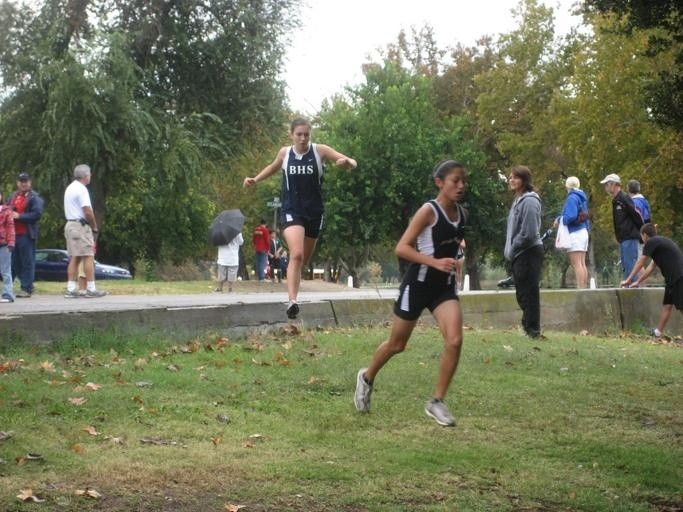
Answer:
[600,173,621,183]
[17,173,30,179]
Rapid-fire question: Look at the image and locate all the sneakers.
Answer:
[64,289,85,298]
[354,368,373,414]
[16,290,31,297]
[85,289,107,297]
[0,293,14,303]
[425,399,455,426]
[647,328,662,337]
[286,300,299,318]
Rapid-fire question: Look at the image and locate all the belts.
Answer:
[67,219,81,221]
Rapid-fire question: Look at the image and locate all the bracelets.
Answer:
[91,228,98,234]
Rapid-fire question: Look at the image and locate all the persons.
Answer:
[619,222,683,337]
[353,158,469,427]
[60,162,109,298]
[252,216,288,284]
[551,175,593,289]
[0,193,17,303]
[501,164,546,342]
[626,176,652,287]
[4,171,45,299]
[76,231,98,295]
[598,172,643,289]
[240,117,361,321]
[214,230,244,294]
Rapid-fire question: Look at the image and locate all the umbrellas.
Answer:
[205,208,244,246]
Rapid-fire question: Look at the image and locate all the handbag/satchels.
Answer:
[573,210,589,224]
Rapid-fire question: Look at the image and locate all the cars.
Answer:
[34,248,131,282]
[498,275,516,287]
